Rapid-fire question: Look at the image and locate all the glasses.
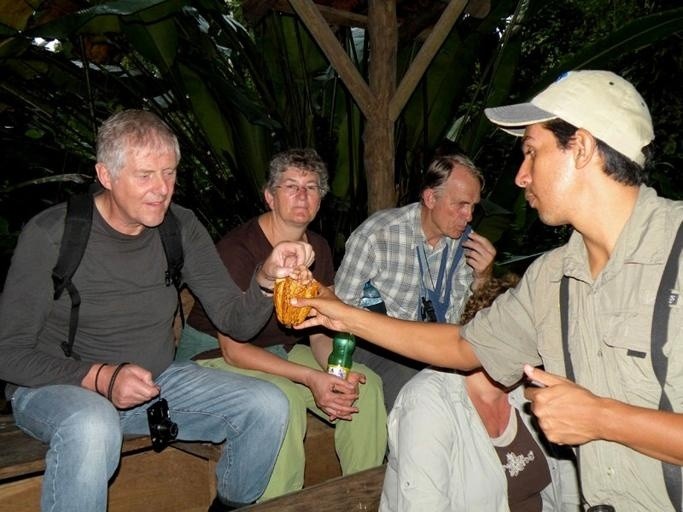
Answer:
[273,184,323,195]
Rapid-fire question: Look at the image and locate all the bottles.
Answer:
[358,282,385,308]
[326,331,357,379]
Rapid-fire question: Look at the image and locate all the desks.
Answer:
[236,461,386,512]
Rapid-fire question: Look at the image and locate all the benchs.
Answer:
[1,409,342,511]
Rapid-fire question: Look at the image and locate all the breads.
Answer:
[274,274,320,325]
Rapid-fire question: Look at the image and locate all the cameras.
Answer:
[147,397,178,452]
[420,297,436,323]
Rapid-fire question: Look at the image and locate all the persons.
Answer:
[0,108,316,512]
[375,271,581,512]
[183,145,390,506]
[289,69,683,512]
[332,151,498,465]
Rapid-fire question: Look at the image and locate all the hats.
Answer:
[483,69,656,170]
